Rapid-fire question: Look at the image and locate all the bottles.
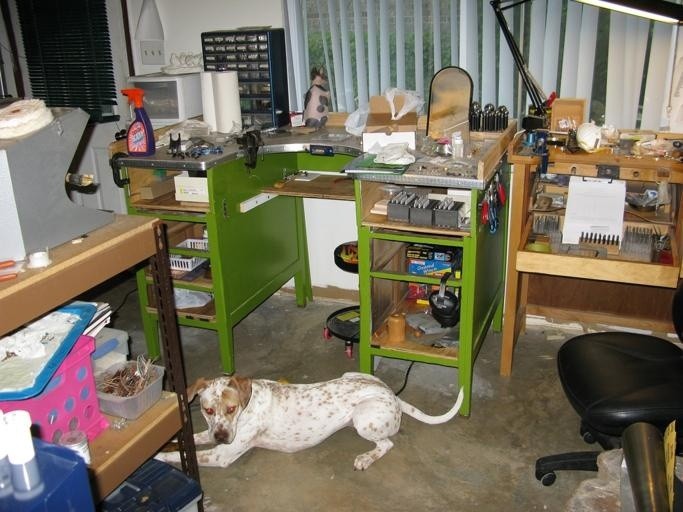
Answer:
[388,313,406,342]
[453,139,464,160]
[0,409,90,501]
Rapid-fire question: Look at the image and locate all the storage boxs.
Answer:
[96,458,203,512]
[0,335,109,449]
[361,96,418,151]
[91,329,129,381]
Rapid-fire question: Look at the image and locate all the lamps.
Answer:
[489,0,683,128]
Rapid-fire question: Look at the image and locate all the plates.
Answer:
[26,260,53,269]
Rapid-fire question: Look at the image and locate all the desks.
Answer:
[110,116,518,418]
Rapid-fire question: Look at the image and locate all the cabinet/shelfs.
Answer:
[127,73,202,130]
[200,28,292,129]
[0,214,206,512]
[499,125,683,378]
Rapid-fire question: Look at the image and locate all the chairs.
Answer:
[535,276,682,486]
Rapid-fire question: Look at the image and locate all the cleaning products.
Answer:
[121,89,155,157]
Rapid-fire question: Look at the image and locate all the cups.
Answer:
[29,252,48,266]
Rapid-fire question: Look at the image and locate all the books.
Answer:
[66,300,113,339]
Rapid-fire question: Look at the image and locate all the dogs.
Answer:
[152,371,463,472]
